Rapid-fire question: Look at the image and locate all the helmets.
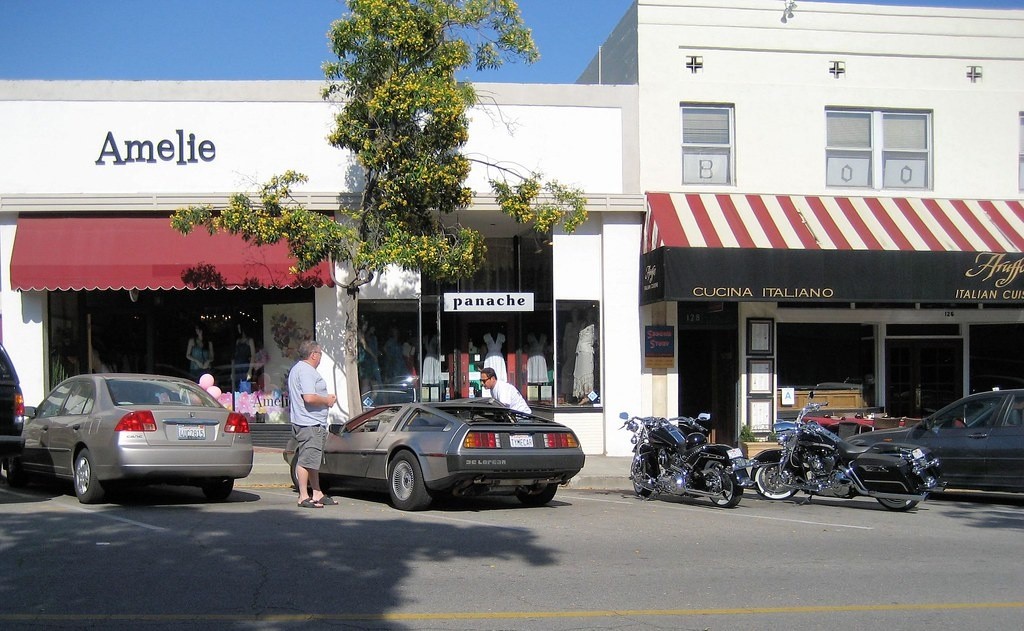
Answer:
[686,432,706,444]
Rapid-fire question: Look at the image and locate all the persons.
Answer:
[288,340,338,509]
[526,307,598,404]
[358,318,382,382]
[231,322,255,387]
[385,326,442,402]
[186,322,214,378]
[480,368,533,414]
[483,331,508,372]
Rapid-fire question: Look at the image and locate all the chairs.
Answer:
[873,417,902,429]
[1008,409,1021,426]
[904,418,922,427]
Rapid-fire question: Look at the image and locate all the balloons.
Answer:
[257,372,278,392]
[190,373,221,406]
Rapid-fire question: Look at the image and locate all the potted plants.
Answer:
[738,423,784,460]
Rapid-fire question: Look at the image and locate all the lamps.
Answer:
[784,0,797,13]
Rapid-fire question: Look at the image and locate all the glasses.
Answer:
[481,377,491,383]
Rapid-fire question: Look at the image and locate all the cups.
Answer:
[256,411,266,423]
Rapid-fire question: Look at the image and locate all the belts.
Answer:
[295,424,320,428]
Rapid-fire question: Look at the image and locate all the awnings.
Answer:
[638,192,1024,306]
[9,213,338,291]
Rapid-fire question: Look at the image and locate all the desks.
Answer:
[802,416,908,427]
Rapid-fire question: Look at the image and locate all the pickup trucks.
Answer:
[0,343,26,461]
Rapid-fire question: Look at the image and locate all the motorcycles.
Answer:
[618,411,758,509]
[746,383,949,512]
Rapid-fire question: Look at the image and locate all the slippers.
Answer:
[298,498,324,508]
[312,495,338,505]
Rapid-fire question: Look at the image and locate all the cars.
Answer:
[842,388,1024,494]
[282,396,587,512]
[4,372,255,504]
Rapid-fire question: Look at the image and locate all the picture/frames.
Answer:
[745,317,774,434]
